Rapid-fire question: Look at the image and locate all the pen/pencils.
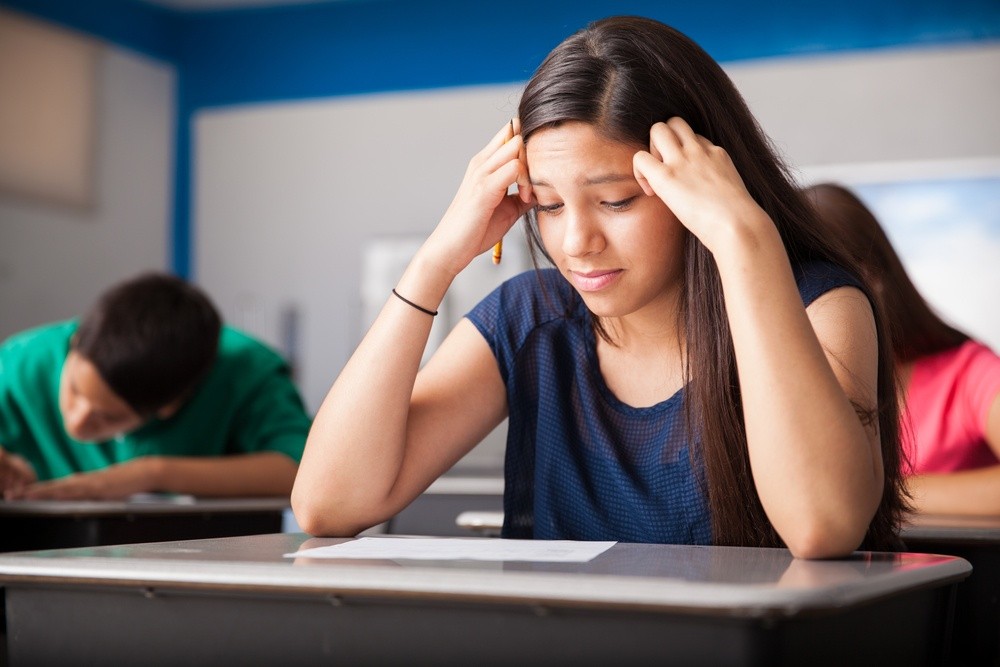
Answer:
[493,118,515,265]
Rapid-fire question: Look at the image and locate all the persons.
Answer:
[294,538,864,591]
[291,16,920,560]
[0,268,316,500]
[801,184,1000,517]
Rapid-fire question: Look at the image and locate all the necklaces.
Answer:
[392,288,439,316]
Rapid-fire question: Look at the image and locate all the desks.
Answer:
[0,466,1000,667]
